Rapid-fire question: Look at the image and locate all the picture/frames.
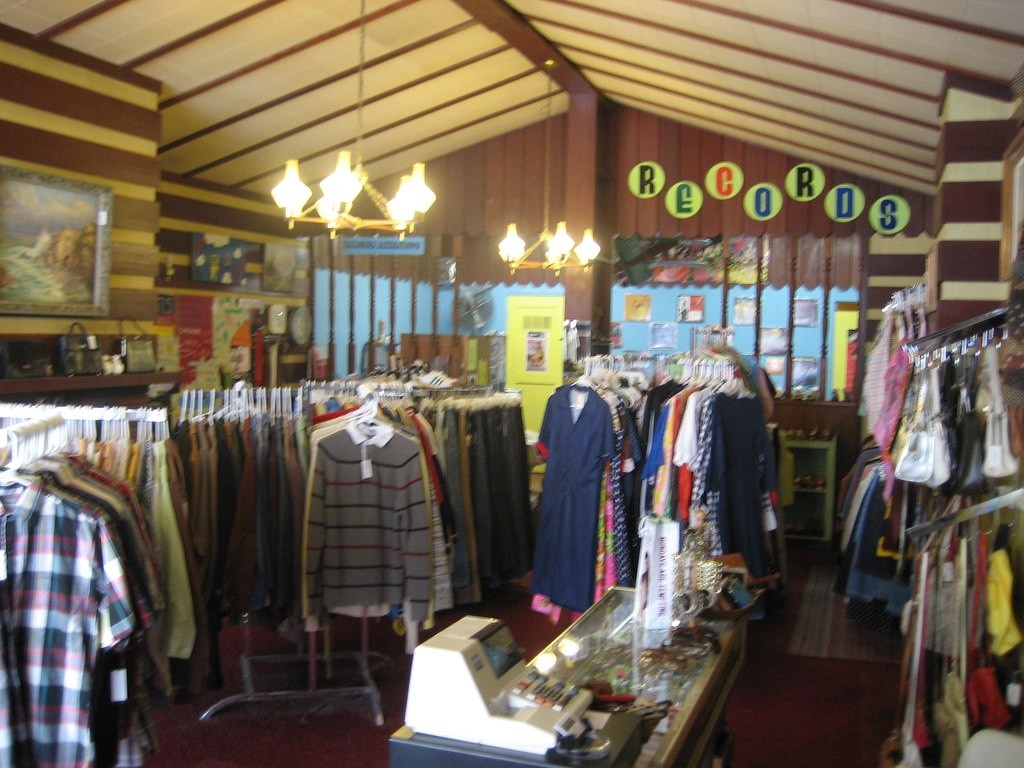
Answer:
[0,164,113,316]
[999,125,1023,287]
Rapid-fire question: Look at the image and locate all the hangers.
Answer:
[0,365,523,492]
[880,283,1010,370]
[571,326,756,399]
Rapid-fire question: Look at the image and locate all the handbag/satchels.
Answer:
[893,344,1024,495]
[881,522,1024,768]
[59,321,103,375]
[119,316,158,374]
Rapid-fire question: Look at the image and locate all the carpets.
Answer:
[788,563,906,663]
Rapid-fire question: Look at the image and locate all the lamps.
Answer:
[498,60,601,277]
[270,0,435,242]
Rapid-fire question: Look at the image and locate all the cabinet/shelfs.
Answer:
[524,586,750,768]
[779,441,839,547]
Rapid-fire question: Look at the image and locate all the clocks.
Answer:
[291,306,313,345]
